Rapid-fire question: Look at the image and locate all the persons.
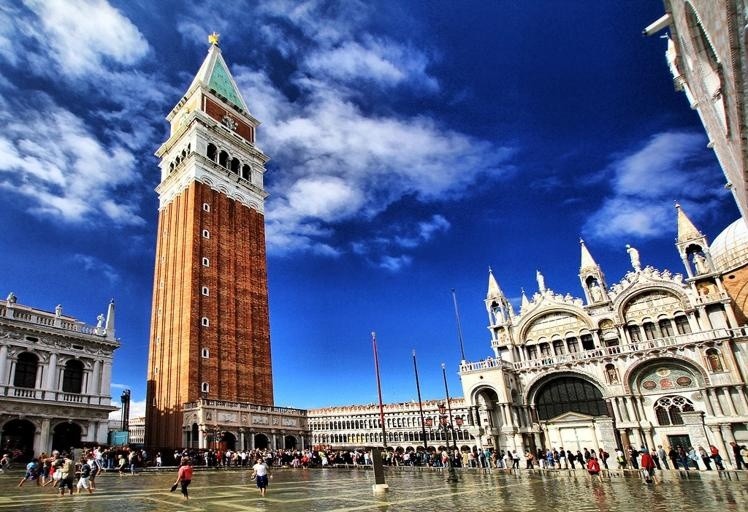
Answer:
[56,304,63,317]
[709,350,725,373]
[590,282,603,302]
[625,244,641,272]
[692,252,708,275]
[536,269,546,296]
[7,292,17,308]
[96,314,105,328]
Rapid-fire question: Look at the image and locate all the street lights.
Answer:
[119,389,128,431]
[426,404,464,483]
